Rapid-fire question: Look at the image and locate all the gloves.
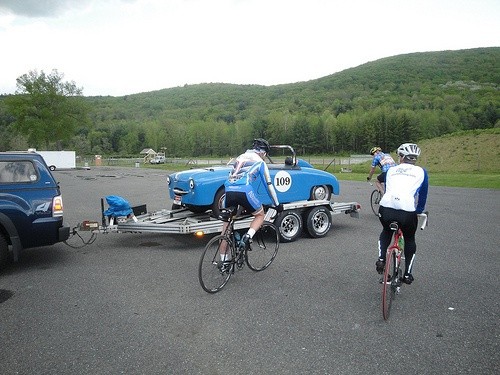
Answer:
[276,203,284,213]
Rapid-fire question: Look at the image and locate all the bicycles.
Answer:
[367,178,383,216]
[379,211,429,320]
[198,206,281,294]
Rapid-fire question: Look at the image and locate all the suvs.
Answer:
[0,152,70,264]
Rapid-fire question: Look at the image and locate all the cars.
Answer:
[150,156,164,164]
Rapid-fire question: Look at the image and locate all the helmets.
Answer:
[253,138,270,152]
[397,143,421,159]
[370,147,382,153]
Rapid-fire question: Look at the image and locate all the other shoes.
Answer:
[222,264,230,271]
[404,275,414,283]
[241,235,253,251]
[375,260,384,274]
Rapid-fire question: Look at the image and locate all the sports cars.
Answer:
[166,144,339,221]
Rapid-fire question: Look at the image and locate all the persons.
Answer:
[367,147,396,196]
[377,144,428,282]
[219,140,284,270]
